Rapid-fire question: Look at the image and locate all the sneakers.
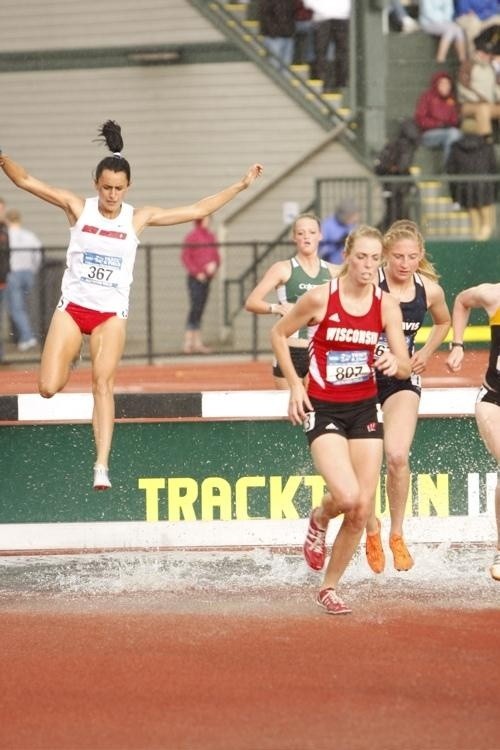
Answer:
[490,550,499,579]
[366,518,385,572]
[315,588,351,615]
[92,466,111,490]
[388,534,414,572]
[305,507,327,570]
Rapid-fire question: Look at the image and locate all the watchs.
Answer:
[448,341,464,351]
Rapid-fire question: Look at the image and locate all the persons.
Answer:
[243,1,350,93]
[0,118,262,489]
[245,213,341,386]
[182,217,220,355]
[446,281,500,581]
[5,209,42,350]
[376,0,500,241]
[360,219,454,573]
[0,201,10,357]
[269,226,412,616]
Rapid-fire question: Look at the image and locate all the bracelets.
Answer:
[267,301,276,314]
